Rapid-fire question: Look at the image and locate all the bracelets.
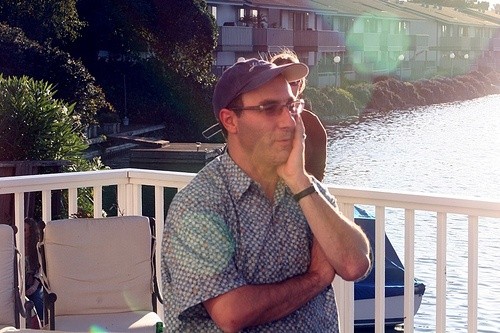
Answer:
[293,183,320,201]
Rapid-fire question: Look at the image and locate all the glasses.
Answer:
[230,98,306,117]
[289,81,300,87]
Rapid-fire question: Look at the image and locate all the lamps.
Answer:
[196,142,201,147]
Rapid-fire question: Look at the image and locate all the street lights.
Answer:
[450,53,455,79]
[398,55,405,80]
[333,56,341,88]
[464,54,469,75]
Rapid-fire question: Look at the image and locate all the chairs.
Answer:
[0,216,163,333]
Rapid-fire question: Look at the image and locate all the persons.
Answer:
[160,58,372,333]
[272,51,326,182]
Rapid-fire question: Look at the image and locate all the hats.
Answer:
[212,58,309,119]
